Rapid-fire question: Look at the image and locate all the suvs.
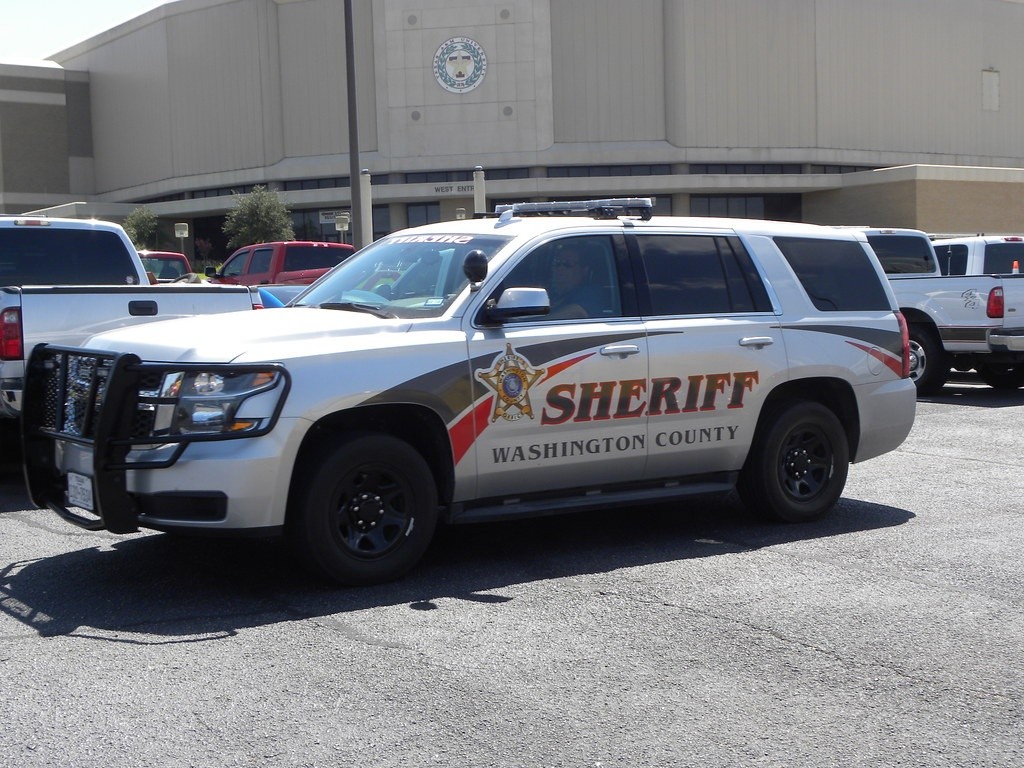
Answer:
[18,199,916,592]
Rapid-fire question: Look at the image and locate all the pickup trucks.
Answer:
[823,225,1024,398]
[0,214,433,464]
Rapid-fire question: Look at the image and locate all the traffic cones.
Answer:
[1010,260,1020,273]
